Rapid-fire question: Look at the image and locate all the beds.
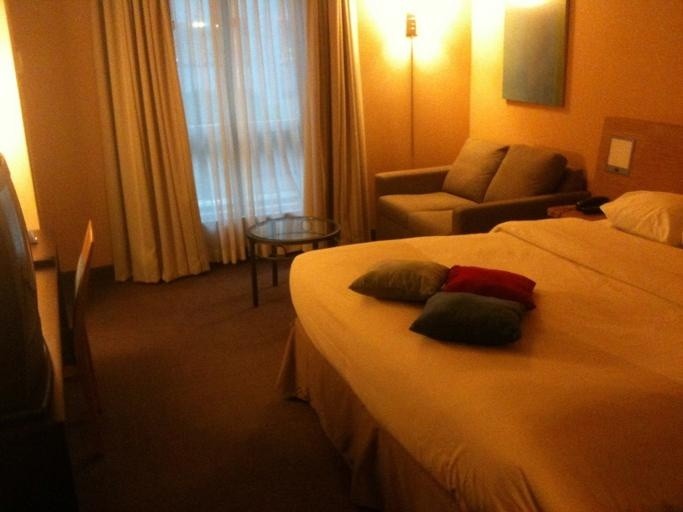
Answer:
[277,116,683,512]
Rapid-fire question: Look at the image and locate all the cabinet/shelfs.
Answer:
[0,223,91,512]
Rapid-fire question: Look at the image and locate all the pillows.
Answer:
[348,262,535,346]
[601,188,683,247]
[443,136,566,205]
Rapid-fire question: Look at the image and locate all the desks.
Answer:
[247,215,341,307]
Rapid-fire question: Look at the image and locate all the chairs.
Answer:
[60,217,99,397]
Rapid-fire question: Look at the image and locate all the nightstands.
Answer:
[545,205,606,221]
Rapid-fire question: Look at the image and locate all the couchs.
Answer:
[374,157,585,237]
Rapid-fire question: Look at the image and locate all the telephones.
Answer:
[576,196,609,214]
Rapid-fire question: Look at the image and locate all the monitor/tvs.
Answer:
[1,152,51,411]
